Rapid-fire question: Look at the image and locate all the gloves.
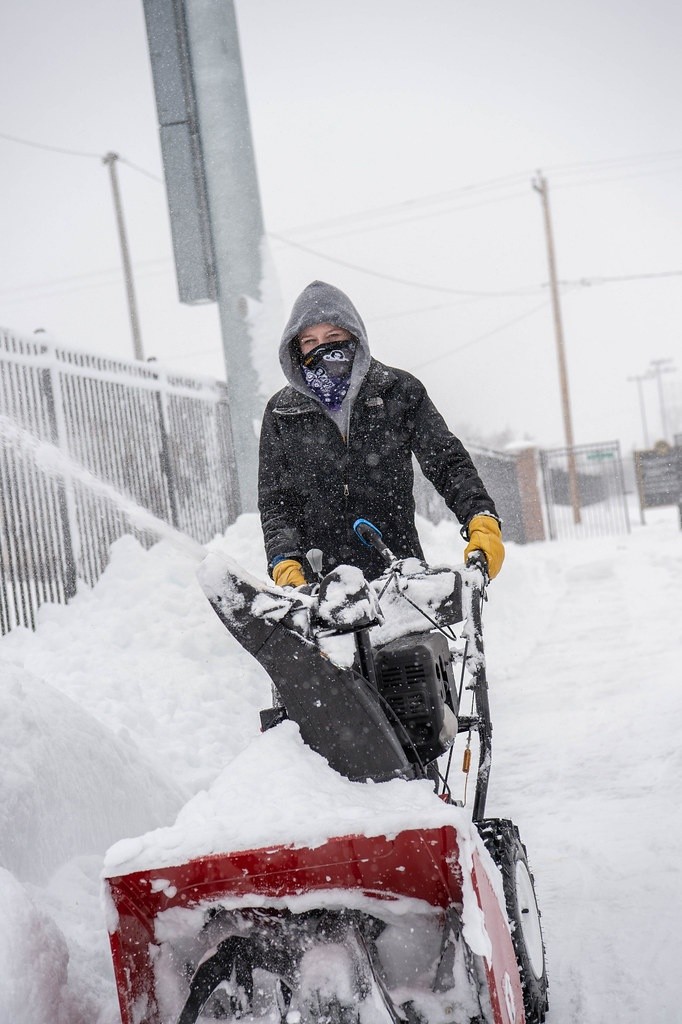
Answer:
[464,514,505,580]
[272,560,305,588]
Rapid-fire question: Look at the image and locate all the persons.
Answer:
[258,279,504,789]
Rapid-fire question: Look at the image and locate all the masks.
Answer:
[302,340,355,377]
